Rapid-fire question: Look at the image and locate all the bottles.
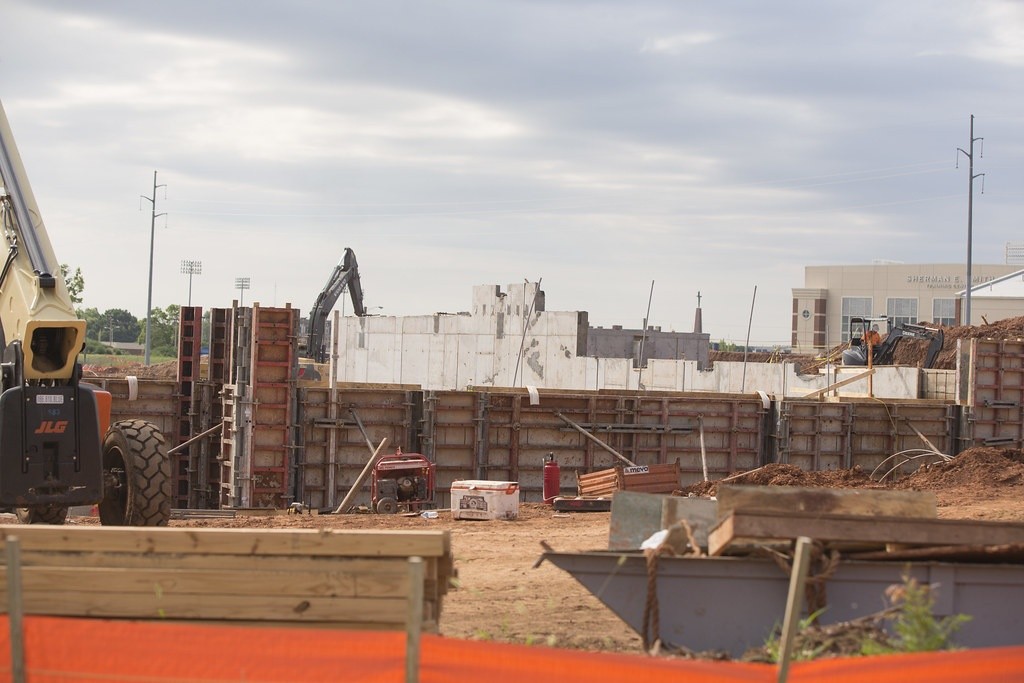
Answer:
[421,512,438,518]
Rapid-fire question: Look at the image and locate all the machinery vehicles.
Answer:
[840,314,944,368]
[297,247,367,381]
[0,99,172,526]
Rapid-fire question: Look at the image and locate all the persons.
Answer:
[861,325,881,346]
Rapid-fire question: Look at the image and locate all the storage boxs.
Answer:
[449,480,519,519]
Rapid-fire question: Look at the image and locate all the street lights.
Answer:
[235,277,251,307]
[954,112,985,327]
[179,260,201,307]
[139,168,168,366]
